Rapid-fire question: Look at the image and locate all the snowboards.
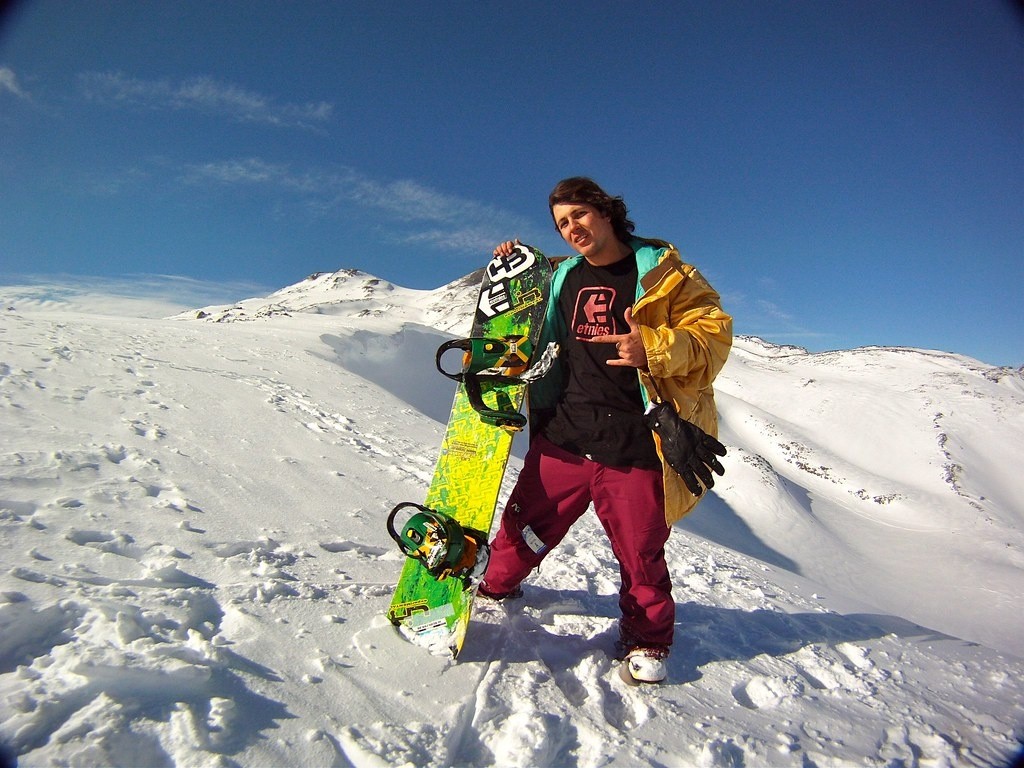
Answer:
[388,245,553,661]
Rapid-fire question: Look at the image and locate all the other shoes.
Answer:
[626,650,667,681]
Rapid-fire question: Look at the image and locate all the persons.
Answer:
[475,178,732,683]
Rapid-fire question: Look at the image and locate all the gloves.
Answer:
[645,401,726,497]
